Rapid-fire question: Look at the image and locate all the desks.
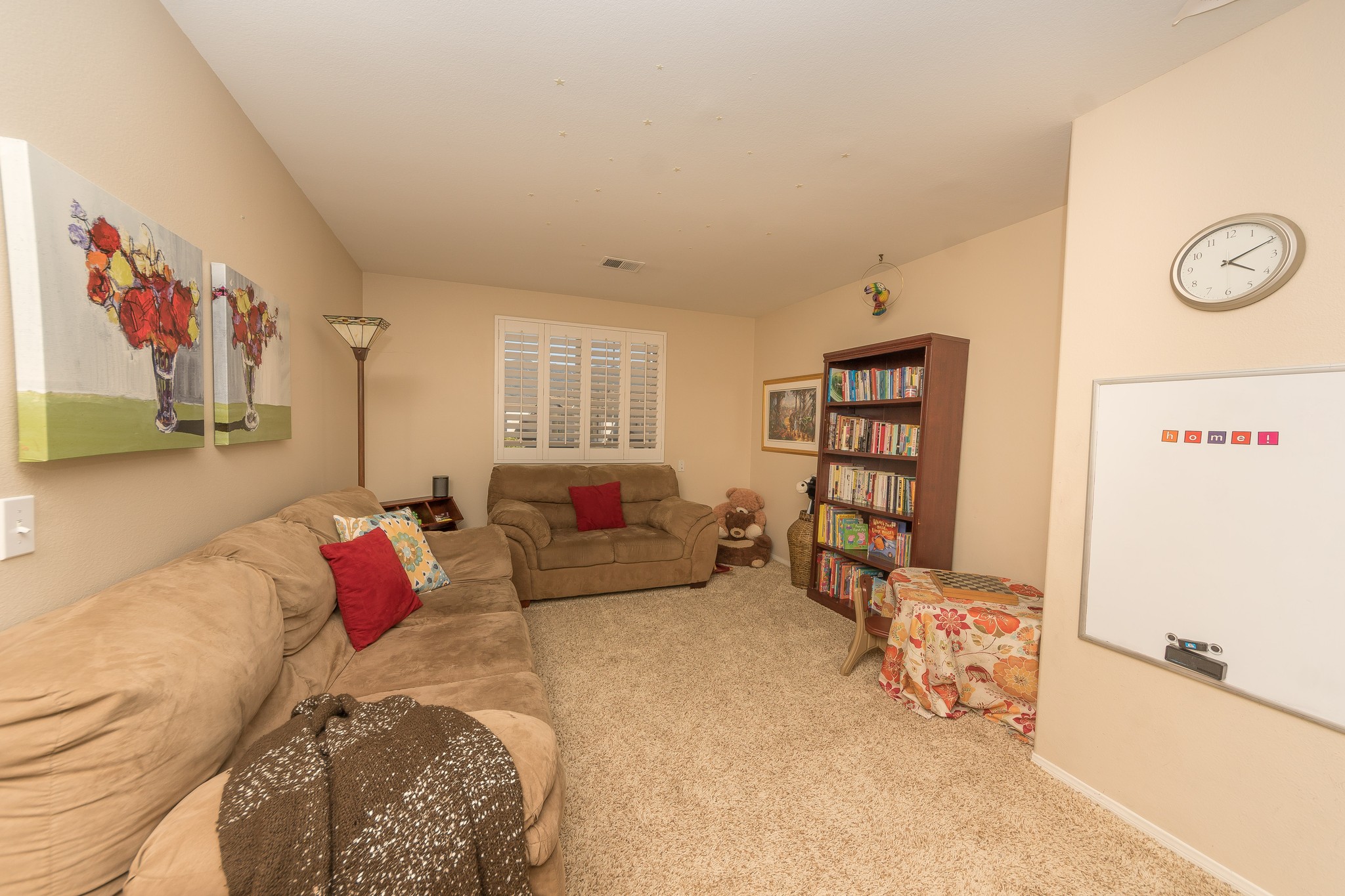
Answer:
[878,567,1044,747]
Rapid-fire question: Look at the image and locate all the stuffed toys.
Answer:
[796,472,817,515]
[715,510,772,568]
[712,488,766,539]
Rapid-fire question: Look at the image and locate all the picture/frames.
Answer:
[761,373,824,457]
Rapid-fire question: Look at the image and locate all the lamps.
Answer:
[324,315,391,486]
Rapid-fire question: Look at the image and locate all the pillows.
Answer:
[568,481,627,532]
[319,526,424,652]
[333,507,452,595]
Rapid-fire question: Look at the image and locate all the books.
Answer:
[813,366,924,614]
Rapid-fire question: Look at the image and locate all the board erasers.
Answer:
[1164,644,1227,681]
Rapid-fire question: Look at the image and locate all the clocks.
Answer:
[1170,212,1306,311]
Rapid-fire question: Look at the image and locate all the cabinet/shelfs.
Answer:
[379,495,464,532]
[806,332,970,621]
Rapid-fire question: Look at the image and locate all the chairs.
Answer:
[840,574,893,676]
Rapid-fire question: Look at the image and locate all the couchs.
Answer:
[487,463,720,608]
[0,486,568,896]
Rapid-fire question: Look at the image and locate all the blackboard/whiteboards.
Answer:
[1077,364,1345,735]
